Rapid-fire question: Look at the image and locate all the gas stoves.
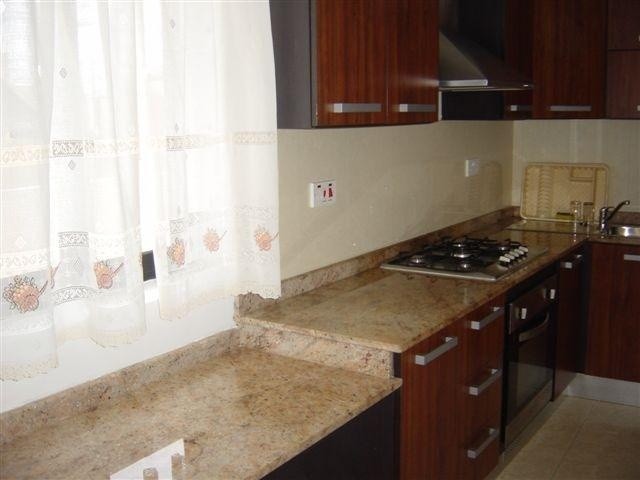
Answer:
[378,235,550,281]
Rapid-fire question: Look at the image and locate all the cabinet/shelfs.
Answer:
[606,0,640,120]
[256,396,399,478]
[557,240,592,402]
[507,0,607,120]
[399,316,469,480]
[583,242,640,406]
[465,297,505,480]
[270,0,438,129]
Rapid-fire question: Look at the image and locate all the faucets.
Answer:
[599,200,630,230]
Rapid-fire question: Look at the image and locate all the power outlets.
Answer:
[466,158,478,177]
[310,179,337,207]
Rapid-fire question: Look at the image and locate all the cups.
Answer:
[569,199,584,239]
[582,203,597,240]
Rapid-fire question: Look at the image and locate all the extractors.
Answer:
[437,0,511,123]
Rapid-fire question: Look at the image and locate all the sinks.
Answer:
[601,225,640,239]
